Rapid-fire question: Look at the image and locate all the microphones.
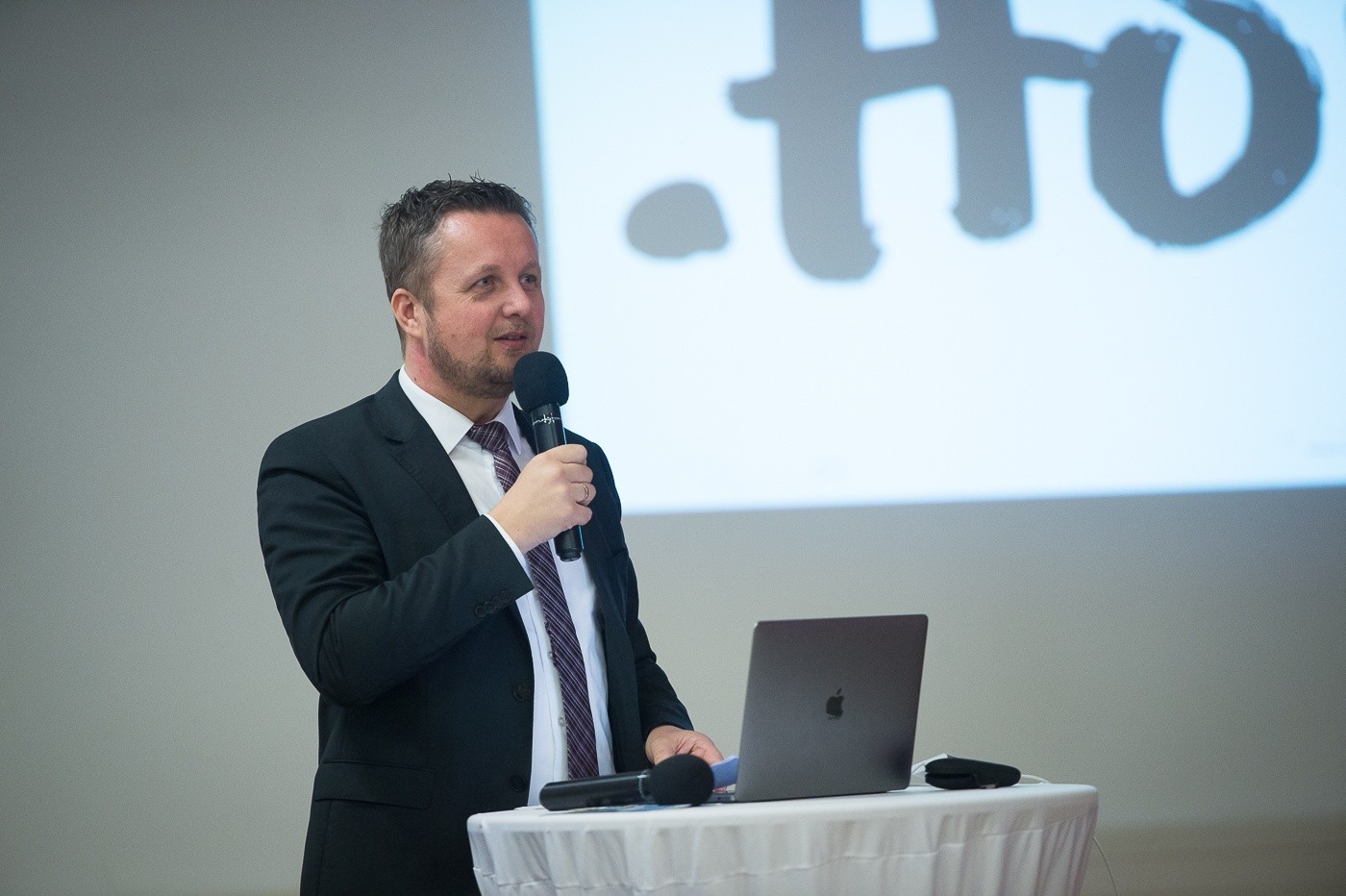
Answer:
[539,753,714,811]
[511,351,588,562]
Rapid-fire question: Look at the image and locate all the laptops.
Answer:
[705,612,929,803]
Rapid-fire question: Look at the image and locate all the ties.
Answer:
[466,421,599,780]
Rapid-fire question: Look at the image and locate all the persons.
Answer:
[257,172,723,896]
